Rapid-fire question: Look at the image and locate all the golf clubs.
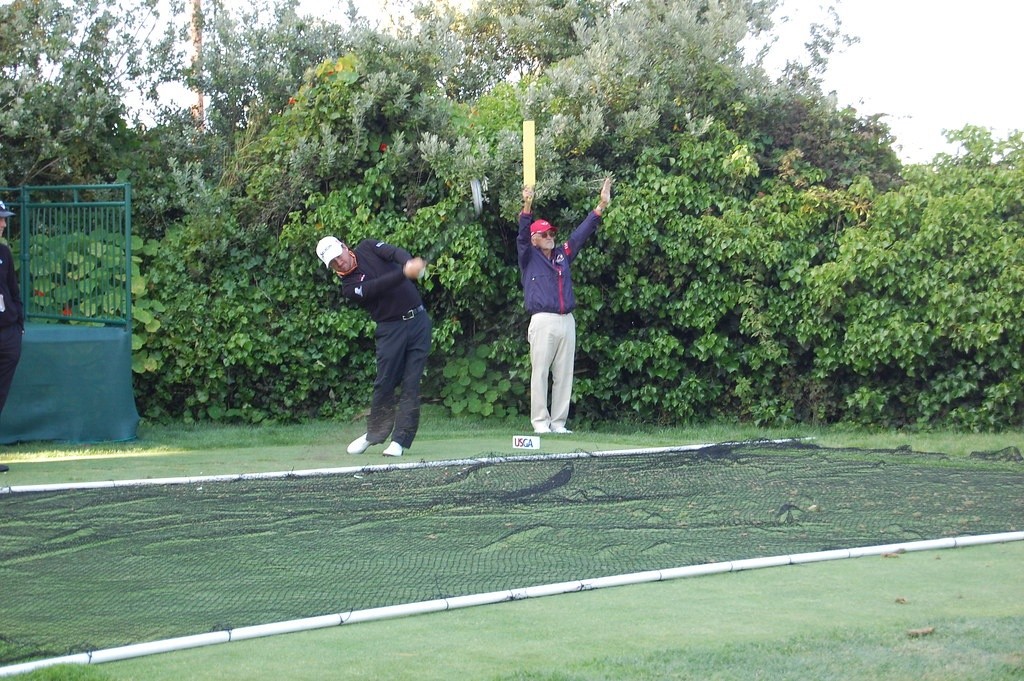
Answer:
[417,176,482,279]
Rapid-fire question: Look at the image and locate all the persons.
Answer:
[517,177,611,434]
[315,235,433,457]
[0,201,24,474]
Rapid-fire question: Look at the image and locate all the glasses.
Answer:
[535,229,556,238]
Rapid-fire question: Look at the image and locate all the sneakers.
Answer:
[346,432,374,454]
[383,440,403,457]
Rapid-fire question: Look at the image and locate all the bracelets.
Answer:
[597,205,605,212]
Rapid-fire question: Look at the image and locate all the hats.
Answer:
[530,219,557,237]
[0,200,16,219]
[315,236,343,269]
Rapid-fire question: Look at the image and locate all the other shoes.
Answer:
[534,428,551,433]
[553,428,572,433]
[0,464,10,472]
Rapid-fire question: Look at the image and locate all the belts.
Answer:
[377,304,425,324]
